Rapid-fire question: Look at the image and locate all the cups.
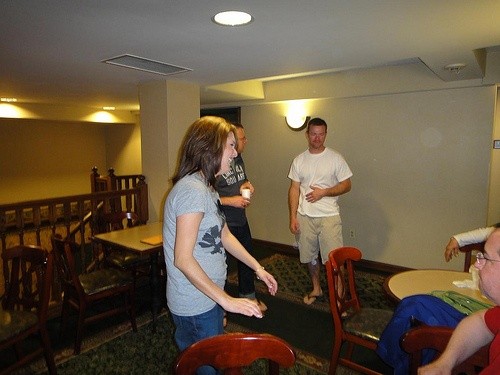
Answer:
[242,189,250,199]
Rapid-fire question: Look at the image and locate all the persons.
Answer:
[288,118,353,303]
[214,123,268,326]
[164,116,278,375]
[417,222,500,375]
[445,223,500,262]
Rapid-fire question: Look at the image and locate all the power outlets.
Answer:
[350,228,355,238]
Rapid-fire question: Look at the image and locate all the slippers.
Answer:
[302,290,325,305]
[340,310,352,319]
[223,309,228,329]
[256,298,269,312]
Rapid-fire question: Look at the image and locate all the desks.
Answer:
[94,222,166,331]
[384,269,498,314]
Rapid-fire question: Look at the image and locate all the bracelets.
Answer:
[257,266,264,279]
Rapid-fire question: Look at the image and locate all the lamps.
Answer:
[285,116,310,131]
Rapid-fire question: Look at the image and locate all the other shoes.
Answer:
[356,357,394,375]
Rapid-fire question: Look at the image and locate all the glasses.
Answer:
[238,136,247,144]
[476,252,500,264]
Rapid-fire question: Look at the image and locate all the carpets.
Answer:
[15,303,364,375]
[226,251,398,316]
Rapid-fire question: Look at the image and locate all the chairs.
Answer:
[401,325,490,375]
[52,234,137,354]
[174,332,295,375]
[100,211,153,278]
[325,248,395,375]
[0,245,58,375]
